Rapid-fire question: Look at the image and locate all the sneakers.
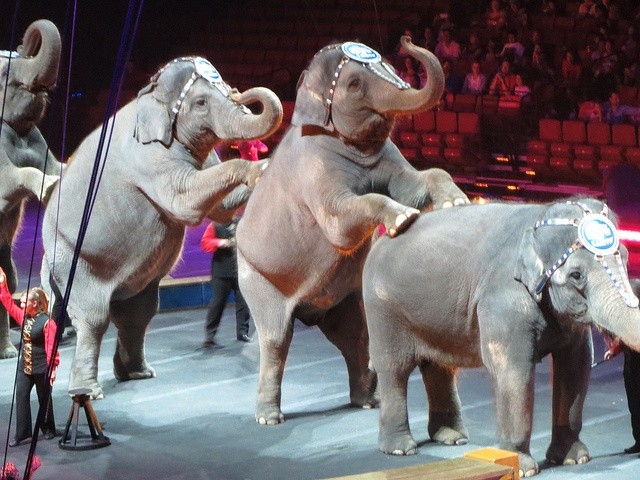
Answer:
[9,433,29,447]
[44,430,55,439]
[237,335,250,343]
[624,441,640,453]
[204,341,224,349]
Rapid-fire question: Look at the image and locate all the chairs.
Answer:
[207,0,392,92]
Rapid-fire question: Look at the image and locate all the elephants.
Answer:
[234,32,472,426]
[361,196,640,479]
[1,16,67,366]
[40,55,283,402]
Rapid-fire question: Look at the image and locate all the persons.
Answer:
[201,216,254,346]
[617,278,640,458]
[0,268,58,446]
[395,0,640,125]
